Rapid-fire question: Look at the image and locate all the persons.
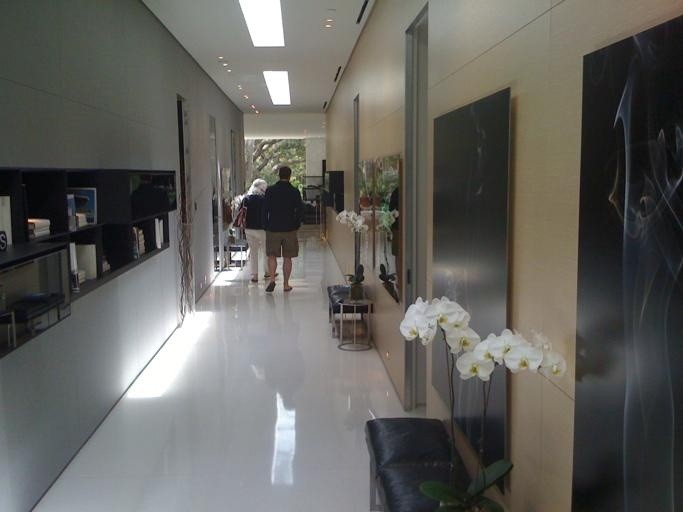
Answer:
[260,165,304,292]
[130,175,168,218]
[239,179,279,283]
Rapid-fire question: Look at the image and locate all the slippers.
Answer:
[266,282,277,291]
[283,285,294,291]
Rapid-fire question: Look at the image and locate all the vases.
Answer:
[382,283,393,296]
[437,494,504,512]
[350,285,363,299]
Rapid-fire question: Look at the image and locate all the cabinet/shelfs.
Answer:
[0,167,176,359]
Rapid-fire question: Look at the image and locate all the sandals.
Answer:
[264,273,279,279]
[252,278,258,282]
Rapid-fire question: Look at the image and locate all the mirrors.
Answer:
[354,152,402,305]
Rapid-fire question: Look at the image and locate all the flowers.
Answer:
[376,209,394,283]
[399,295,567,512]
[336,210,372,284]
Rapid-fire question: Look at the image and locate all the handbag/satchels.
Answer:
[233,205,248,228]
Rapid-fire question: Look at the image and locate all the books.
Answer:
[133,216,164,259]
[66,187,96,293]
[29,230,52,240]
[26,218,51,229]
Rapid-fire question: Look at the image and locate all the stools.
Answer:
[326,285,374,340]
[375,467,483,512]
[336,298,374,351]
[229,241,248,265]
[364,417,459,512]
